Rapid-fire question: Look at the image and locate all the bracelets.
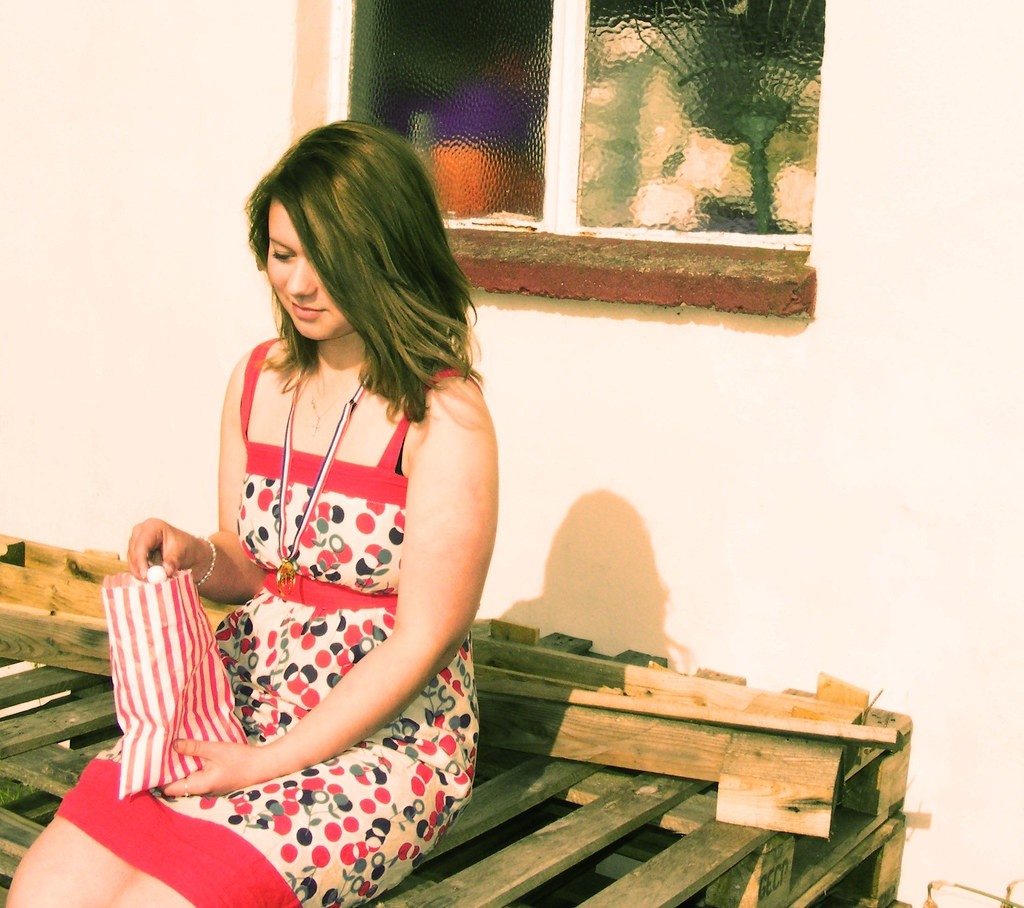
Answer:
[193,533,217,590]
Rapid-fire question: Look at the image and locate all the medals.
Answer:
[278,563,294,588]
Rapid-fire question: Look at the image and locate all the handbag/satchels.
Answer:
[104,571,248,800]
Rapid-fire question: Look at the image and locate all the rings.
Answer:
[184,779,192,799]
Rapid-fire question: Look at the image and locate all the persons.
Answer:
[6,120,500,908]
